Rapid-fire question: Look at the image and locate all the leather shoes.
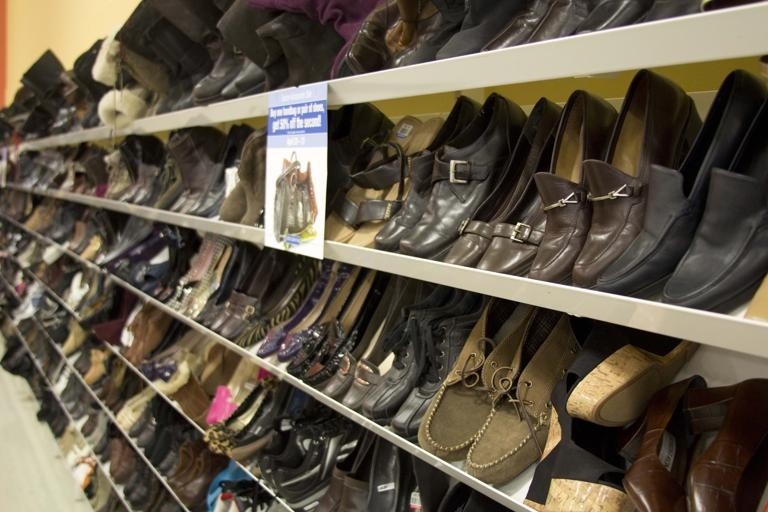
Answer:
[0,69,764,511]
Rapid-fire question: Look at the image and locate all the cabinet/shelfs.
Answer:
[1,4,768,512]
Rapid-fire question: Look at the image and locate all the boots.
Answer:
[0,2,764,141]
